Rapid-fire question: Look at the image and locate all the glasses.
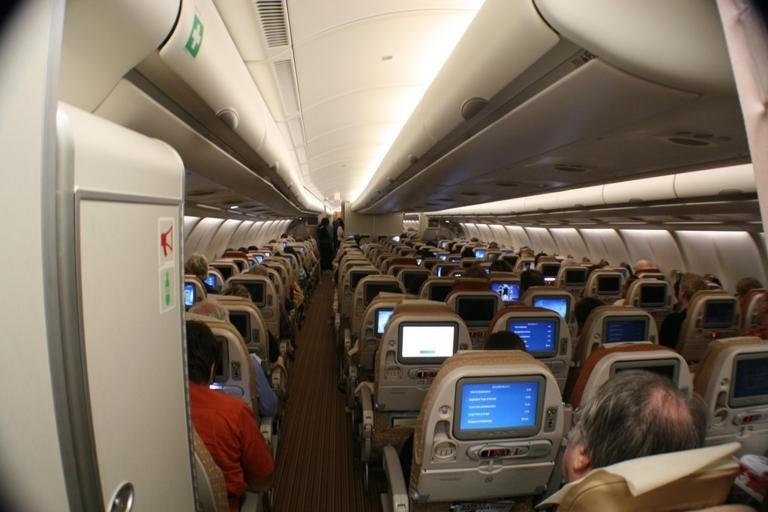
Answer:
[572,407,583,425]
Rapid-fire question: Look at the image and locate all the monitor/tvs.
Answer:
[453,377,545,441]
[604,316,648,342]
[342,237,587,301]
[428,219,439,227]
[705,300,736,328]
[609,359,679,390]
[597,262,669,308]
[183,237,309,382]
[457,296,498,326]
[507,316,560,357]
[399,322,458,364]
[374,309,394,336]
[533,295,570,323]
[308,216,318,225]
[730,354,768,407]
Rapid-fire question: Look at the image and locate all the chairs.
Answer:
[177,219,323,512]
[333,227,766,512]
